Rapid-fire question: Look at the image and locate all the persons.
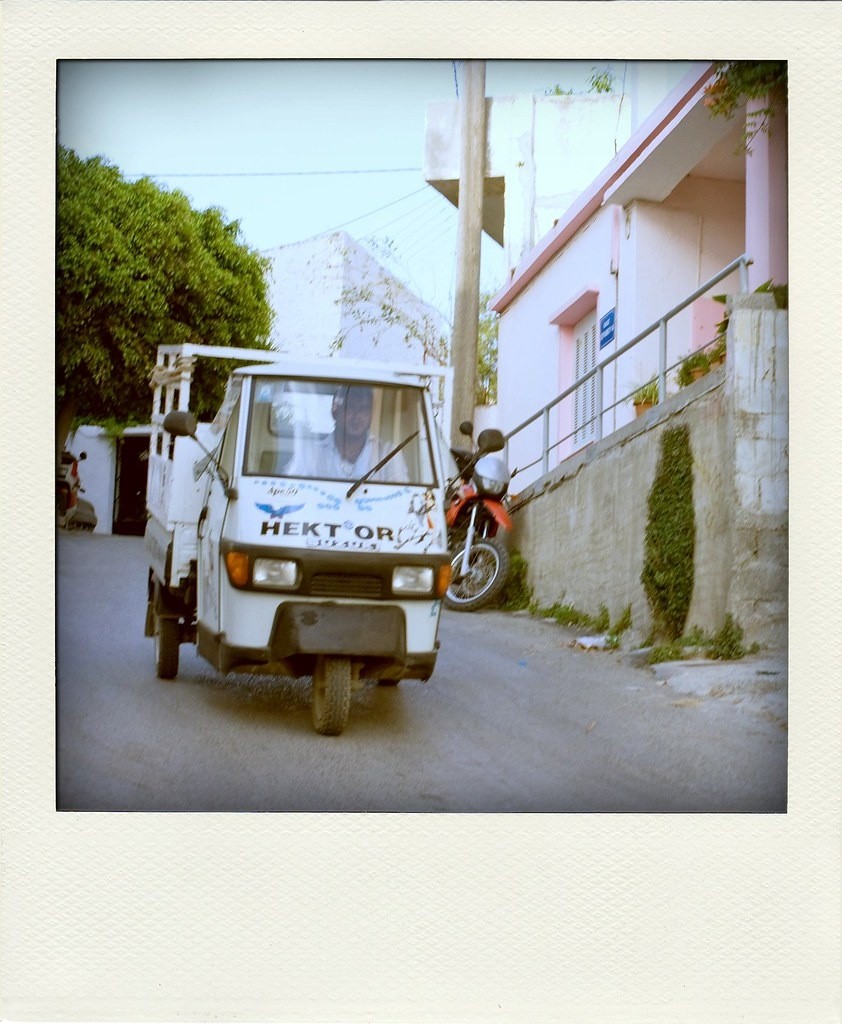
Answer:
[280,383,409,484]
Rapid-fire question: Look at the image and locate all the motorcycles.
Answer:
[441,422,517,614]
[56,453,87,531]
[145,342,455,735]
